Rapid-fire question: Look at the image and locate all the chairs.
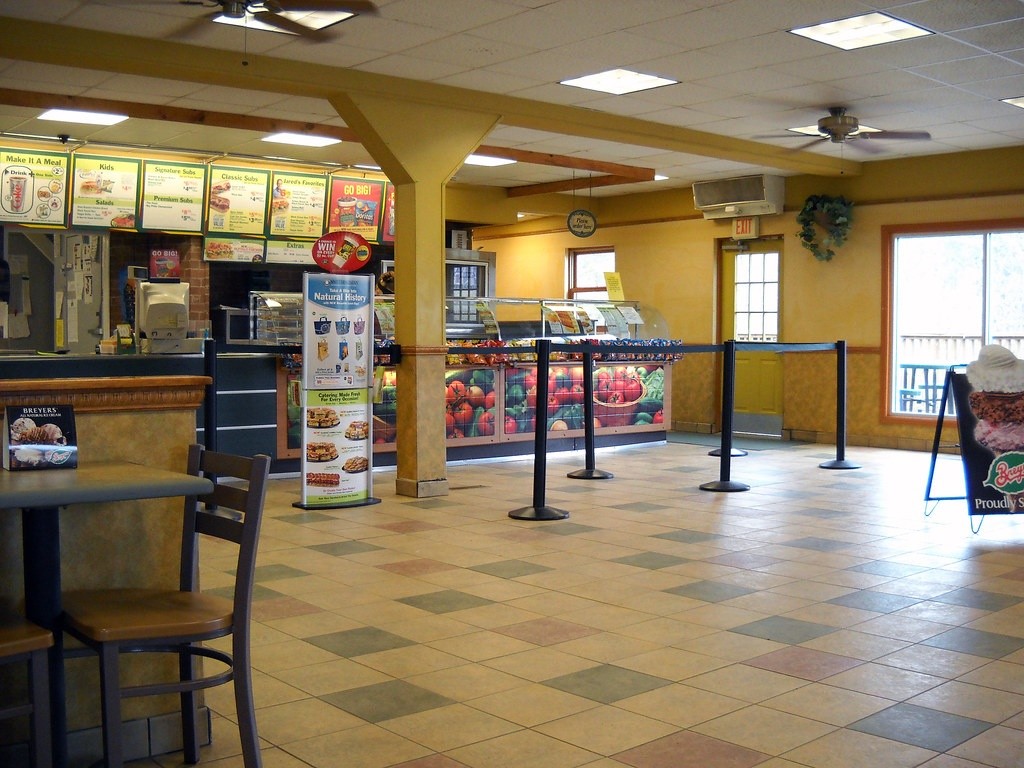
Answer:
[2,596,54,768]
[49,440,272,768]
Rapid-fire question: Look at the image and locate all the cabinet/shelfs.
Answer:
[248,290,674,363]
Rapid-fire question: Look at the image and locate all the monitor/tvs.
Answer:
[140,282,190,339]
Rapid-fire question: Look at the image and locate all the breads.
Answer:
[306,407,369,488]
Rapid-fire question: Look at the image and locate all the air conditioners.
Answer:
[692,174,786,220]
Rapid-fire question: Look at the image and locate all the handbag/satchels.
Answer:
[314,317,331,335]
[354,317,365,335]
[335,317,350,335]
[355,339,363,361]
[318,339,330,362]
[339,338,349,360]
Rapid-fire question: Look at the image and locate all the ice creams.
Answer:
[965,343,1023,513]
[9,418,67,468]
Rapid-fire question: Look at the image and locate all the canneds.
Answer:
[8,176,28,212]
[154,257,171,277]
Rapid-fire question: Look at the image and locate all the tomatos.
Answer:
[504,364,585,434]
[598,367,640,405]
[445,380,519,438]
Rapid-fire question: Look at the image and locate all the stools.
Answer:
[899,385,921,413]
[918,383,948,414]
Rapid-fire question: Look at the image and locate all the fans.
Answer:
[162,0,380,66]
[751,106,930,174]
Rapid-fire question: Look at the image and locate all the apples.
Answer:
[652,407,664,423]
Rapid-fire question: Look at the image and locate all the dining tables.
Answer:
[900,363,970,415]
[1,461,215,767]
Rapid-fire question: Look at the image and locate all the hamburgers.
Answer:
[80,179,101,195]
[206,241,232,259]
[210,178,231,213]
